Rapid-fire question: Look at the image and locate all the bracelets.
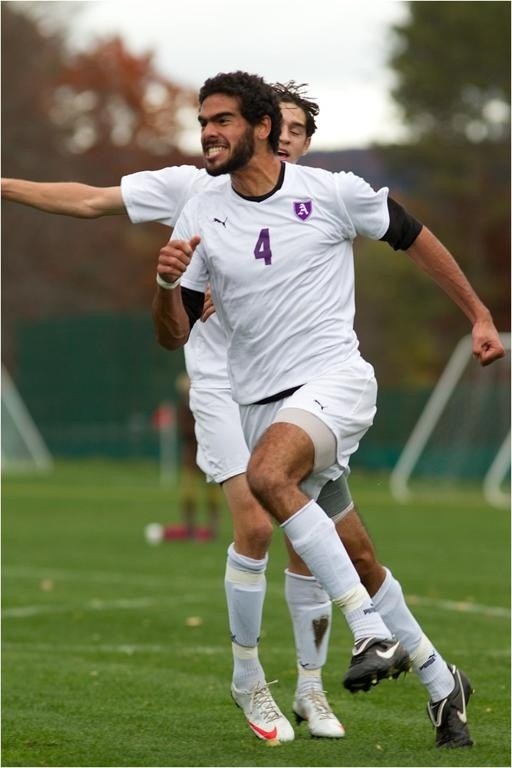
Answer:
[156,273,183,292]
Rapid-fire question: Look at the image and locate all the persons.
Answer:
[155,68,507,753]
[1,76,349,748]
[159,365,222,542]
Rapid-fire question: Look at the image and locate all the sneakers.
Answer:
[230,683,295,744]
[426,662,475,749]
[344,637,412,693]
[291,690,346,739]
